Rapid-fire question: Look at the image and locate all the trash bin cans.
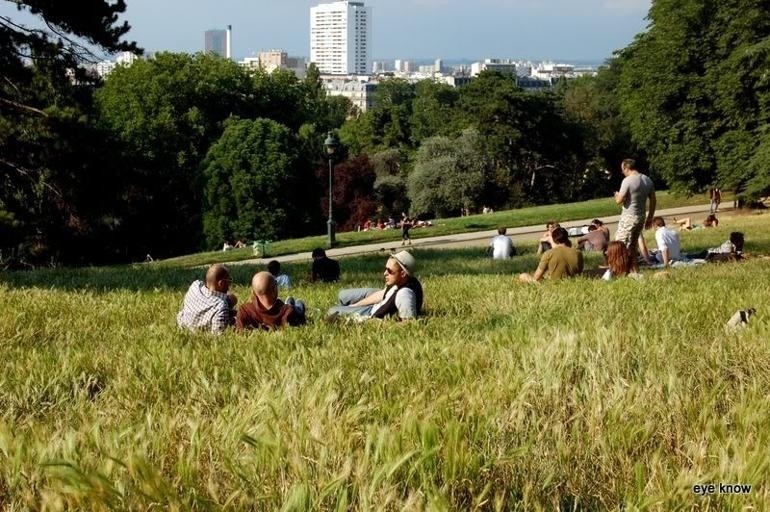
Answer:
[252,239,274,257]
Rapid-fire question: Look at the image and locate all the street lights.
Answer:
[322,130,342,249]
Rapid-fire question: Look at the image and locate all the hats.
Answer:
[391,250,417,278]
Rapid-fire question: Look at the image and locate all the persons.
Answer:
[484,225,518,260]
[364,211,432,245]
[223,241,231,252]
[327,251,425,327]
[235,240,246,248]
[482,206,493,214]
[178,265,237,335]
[267,260,290,288]
[235,271,306,332]
[519,158,747,283]
[309,247,340,282]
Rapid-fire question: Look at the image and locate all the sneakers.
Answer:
[406,242,412,245]
[294,299,305,317]
[401,242,405,246]
[673,217,678,224]
[284,296,295,312]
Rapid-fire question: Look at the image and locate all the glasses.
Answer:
[384,268,394,274]
